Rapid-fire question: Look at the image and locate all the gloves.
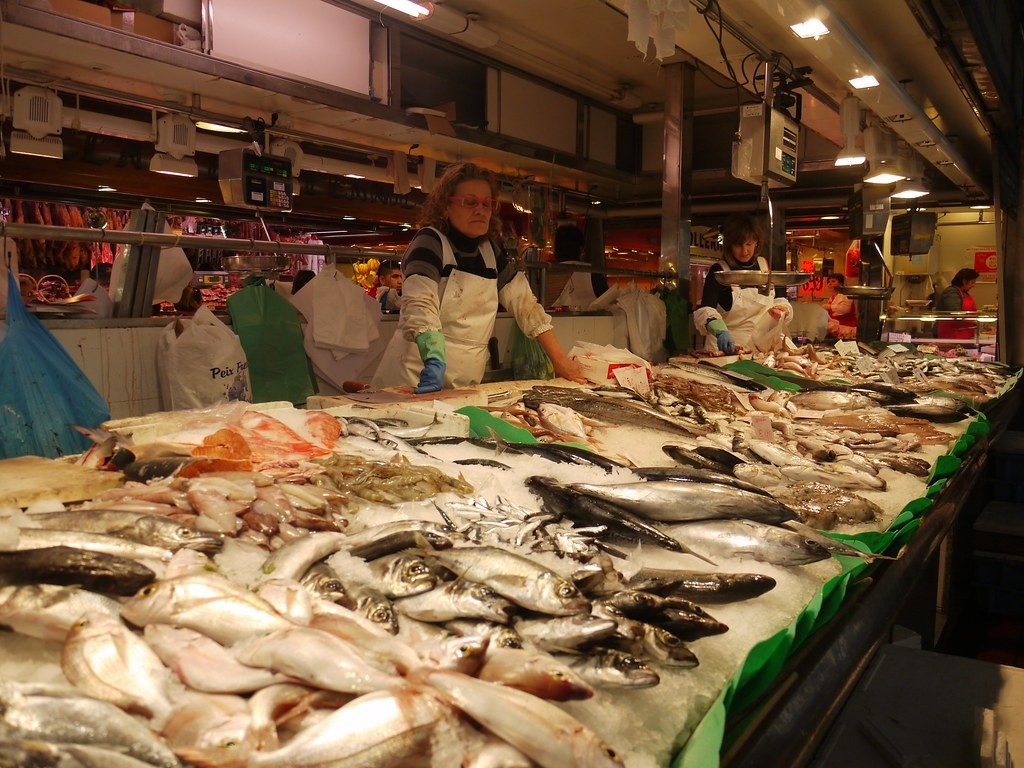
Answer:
[415,331,446,393]
[708,319,736,355]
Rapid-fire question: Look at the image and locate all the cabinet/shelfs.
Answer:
[882,308,998,353]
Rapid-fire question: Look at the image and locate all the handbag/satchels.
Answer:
[156,305,253,412]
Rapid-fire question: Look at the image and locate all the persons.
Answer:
[368,160,588,393]
[92,262,114,293]
[368,260,403,310]
[931,268,980,340]
[173,286,202,316]
[820,275,857,340]
[693,214,793,354]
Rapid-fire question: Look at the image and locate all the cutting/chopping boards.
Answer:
[0,454,126,510]
[669,355,754,368]
[306,384,490,414]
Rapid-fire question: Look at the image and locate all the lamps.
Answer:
[150,109,198,177]
[371,0,642,111]
[8,85,65,161]
[834,91,929,199]
[269,138,302,195]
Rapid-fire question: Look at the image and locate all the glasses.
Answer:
[448,195,498,211]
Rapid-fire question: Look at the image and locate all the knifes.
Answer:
[344,379,414,404]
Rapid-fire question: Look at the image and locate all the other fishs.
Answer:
[0,334,1016,767]
